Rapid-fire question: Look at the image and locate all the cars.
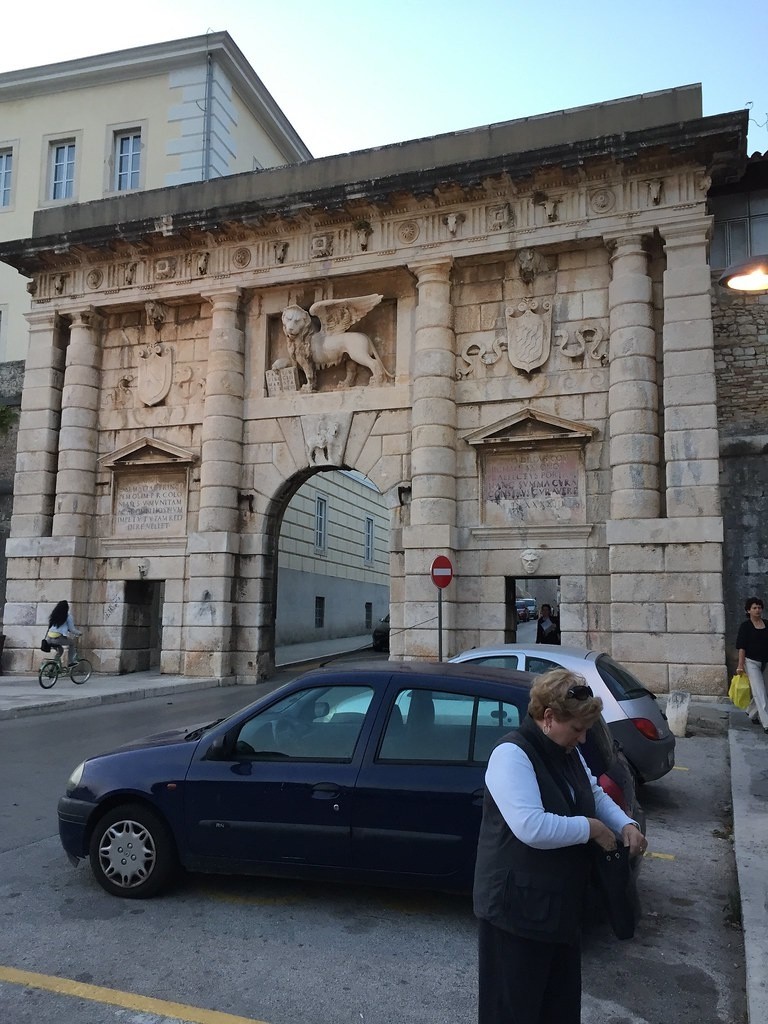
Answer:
[58,660,647,900]
[516,599,539,624]
[373,614,389,649]
[313,644,676,785]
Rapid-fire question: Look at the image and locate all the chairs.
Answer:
[378,704,404,761]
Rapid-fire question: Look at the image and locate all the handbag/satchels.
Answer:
[41,639,52,652]
[728,672,751,709]
[591,830,642,940]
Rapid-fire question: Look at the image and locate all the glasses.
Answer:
[567,685,594,700]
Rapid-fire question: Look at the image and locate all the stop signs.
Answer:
[430,556,453,588]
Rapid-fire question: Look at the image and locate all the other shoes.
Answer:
[751,717,761,725]
[68,662,80,669]
[764,729,768,734]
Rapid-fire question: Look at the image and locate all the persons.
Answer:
[536,603,561,646]
[737,597,768,735]
[40,600,83,676]
[473,670,648,1024]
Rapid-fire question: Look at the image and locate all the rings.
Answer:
[640,846,643,853]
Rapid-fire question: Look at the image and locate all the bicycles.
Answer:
[37,631,93,689]
